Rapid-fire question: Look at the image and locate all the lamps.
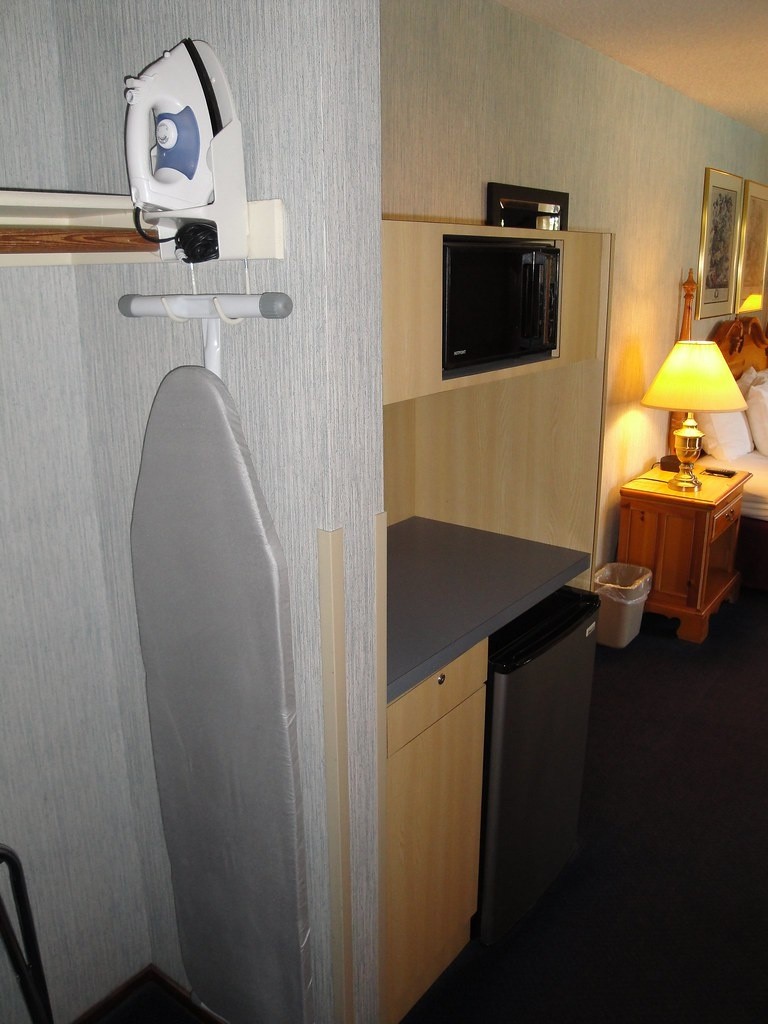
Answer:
[746,380,768,458]
[638,339,748,495]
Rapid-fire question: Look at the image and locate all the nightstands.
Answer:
[619,459,753,646]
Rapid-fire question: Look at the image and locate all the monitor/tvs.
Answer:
[441,233,566,383]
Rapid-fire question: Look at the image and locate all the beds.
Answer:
[662,268,768,524]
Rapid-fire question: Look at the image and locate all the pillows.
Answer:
[686,366,760,459]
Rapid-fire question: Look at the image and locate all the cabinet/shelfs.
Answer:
[370,635,500,1023]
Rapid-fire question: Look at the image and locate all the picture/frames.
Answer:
[693,165,746,321]
[732,178,768,315]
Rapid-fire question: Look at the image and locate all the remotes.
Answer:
[705,467,735,476]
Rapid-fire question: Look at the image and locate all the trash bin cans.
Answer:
[594,562,652,650]
